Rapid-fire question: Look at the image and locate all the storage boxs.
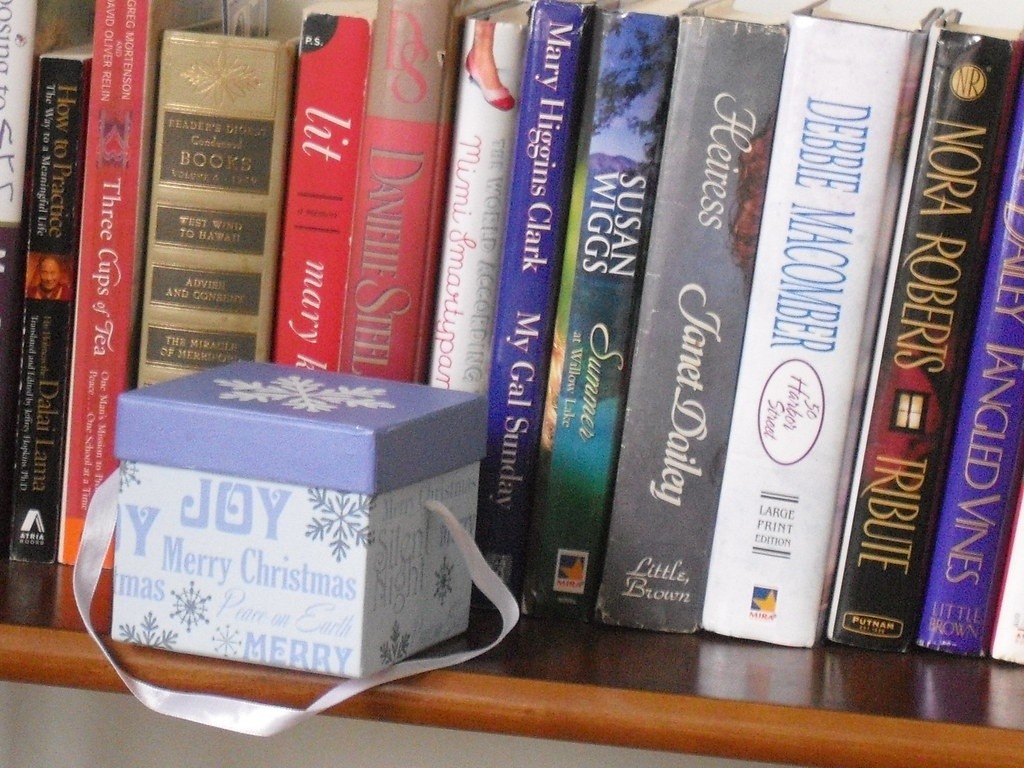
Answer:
[112,359,490,679]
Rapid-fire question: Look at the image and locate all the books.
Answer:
[0,0,1024,665]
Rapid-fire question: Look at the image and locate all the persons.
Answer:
[465,20,516,112]
[27,257,73,301]
